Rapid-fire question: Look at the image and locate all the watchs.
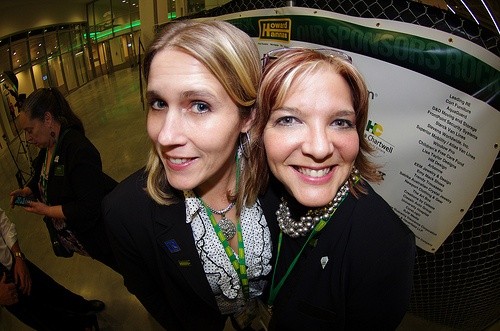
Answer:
[12,252,24,260]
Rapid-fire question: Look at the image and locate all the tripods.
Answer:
[2,134,31,188]
[8,106,29,161]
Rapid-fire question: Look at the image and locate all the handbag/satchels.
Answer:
[43,216,75,258]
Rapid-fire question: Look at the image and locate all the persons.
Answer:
[0,205,106,331]
[104,19,270,331]
[9,88,130,257]
[258,49,417,329]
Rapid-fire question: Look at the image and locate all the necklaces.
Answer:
[277,170,358,236]
[192,190,245,241]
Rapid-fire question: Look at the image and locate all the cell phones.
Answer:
[14,196,38,207]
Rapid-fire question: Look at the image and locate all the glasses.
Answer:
[261,47,353,73]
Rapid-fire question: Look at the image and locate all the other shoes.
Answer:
[89,300,105,312]
